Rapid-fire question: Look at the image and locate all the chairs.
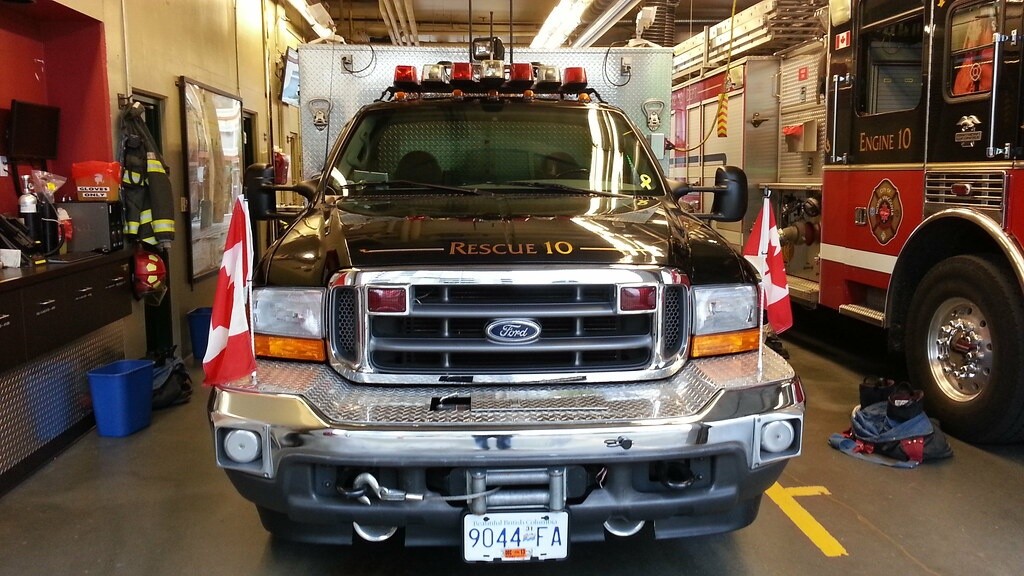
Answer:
[452,169,484,185]
[392,150,449,195]
[544,152,588,180]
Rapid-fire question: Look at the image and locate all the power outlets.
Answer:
[621,57,632,76]
[340,56,353,74]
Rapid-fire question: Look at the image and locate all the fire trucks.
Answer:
[671,1,1024,434]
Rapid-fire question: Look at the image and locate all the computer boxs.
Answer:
[53,201,124,252]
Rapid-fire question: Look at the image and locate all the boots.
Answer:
[857,374,954,460]
[137,344,193,408]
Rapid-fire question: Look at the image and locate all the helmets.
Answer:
[132,252,167,296]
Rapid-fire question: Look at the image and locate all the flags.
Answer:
[200,198,258,387]
[741,198,793,334]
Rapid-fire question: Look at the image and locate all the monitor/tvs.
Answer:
[6,99,60,159]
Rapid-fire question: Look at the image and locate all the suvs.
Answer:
[203,36,808,569]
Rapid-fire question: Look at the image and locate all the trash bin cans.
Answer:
[83,358,157,437]
[187,307,213,360]
